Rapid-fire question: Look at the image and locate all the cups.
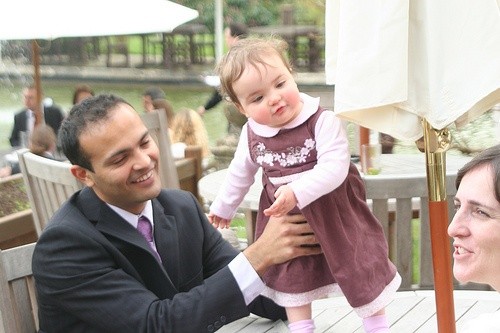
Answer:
[362,144,381,175]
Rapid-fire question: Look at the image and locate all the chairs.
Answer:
[0,242,39,333]
[362,174,457,291]
[16,148,85,238]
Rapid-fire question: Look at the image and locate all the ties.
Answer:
[137,216,162,263]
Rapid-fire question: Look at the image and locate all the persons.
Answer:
[32,94,322,333]
[10,21,245,175]
[447,143,500,294]
[209,32,403,333]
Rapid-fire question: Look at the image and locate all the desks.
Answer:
[212,289,500,333]
[198,154,474,246]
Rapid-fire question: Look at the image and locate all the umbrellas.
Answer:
[0,0,199,126]
[324,0,500,333]
